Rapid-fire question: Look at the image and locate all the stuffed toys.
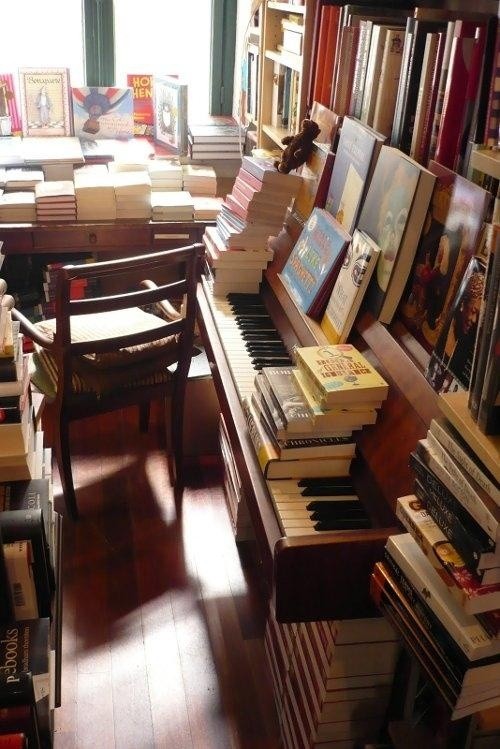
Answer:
[274,119,322,174]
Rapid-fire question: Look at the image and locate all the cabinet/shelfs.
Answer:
[259,0,310,158]
[266,1,500,527]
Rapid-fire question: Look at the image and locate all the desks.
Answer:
[0,214,219,253]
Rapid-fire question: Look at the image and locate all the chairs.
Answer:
[9,241,202,522]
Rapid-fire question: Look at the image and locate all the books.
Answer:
[74,165,117,221]
[277,13,304,62]
[426,21,456,166]
[418,439,492,537]
[387,532,500,661]
[47,253,95,269]
[254,375,355,439]
[468,226,500,425]
[12,321,19,359]
[319,229,381,345]
[2,479,51,560]
[1,294,15,310]
[296,344,389,402]
[127,74,155,137]
[216,215,269,248]
[235,177,291,206]
[37,203,76,209]
[382,546,500,672]
[5,167,44,190]
[149,156,183,190]
[192,194,226,221]
[409,451,500,568]
[220,204,282,235]
[183,164,217,193]
[187,116,245,143]
[397,160,491,354]
[371,562,452,672]
[262,367,362,431]
[251,392,356,460]
[0,357,44,480]
[434,20,476,163]
[38,267,99,321]
[37,209,76,214]
[241,397,352,480]
[347,19,366,117]
[187,135,246,153]
[187,150,244,160]
[202,264,260,294]
[226,194,285,223]
[420,32,447,164]
[36,180,76,202]
[411,479,500,585]
[290,370,377,426]
[427,430,499,535]
[233,186,286,214]
[1,306,13,359]
[152,74,188,154]
[238,167,298,196]
[116,150,147,171]
[487,17,499,149]
[430,417,499,506]
[409,33,439,161]
[313,6,400,113]
[241,155,301,186]
[1,192,37,222]
[204,248,263,282]
[454,27,497,171]
[360,25,405,128]
[281,208,351,318]
[282,64,299,136]
[357,146,438,325]
[82,138,156,159]
[285,101,344,242]
[303,375,384,410]
[1,509,55,621]
[151,191,195,221]
[390,7,485,147]
[0,278,6,295]
[38,215,75,221]
[396,494,499,614]
[206,227,274,261]
[325,116,387,235]
[294,141,336,215]
[72,87,134,140]
[248,54,258,120]
[107,161,152,221]
[477,294,500,432]
[0,618,55,749]
[271,60,285,129]
[373,29,405,138]
[201,234,267,269]
[355,19,406,119]
[22,137,85,164]
[425,222,493,395]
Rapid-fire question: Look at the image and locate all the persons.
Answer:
[36,86,50,125]
